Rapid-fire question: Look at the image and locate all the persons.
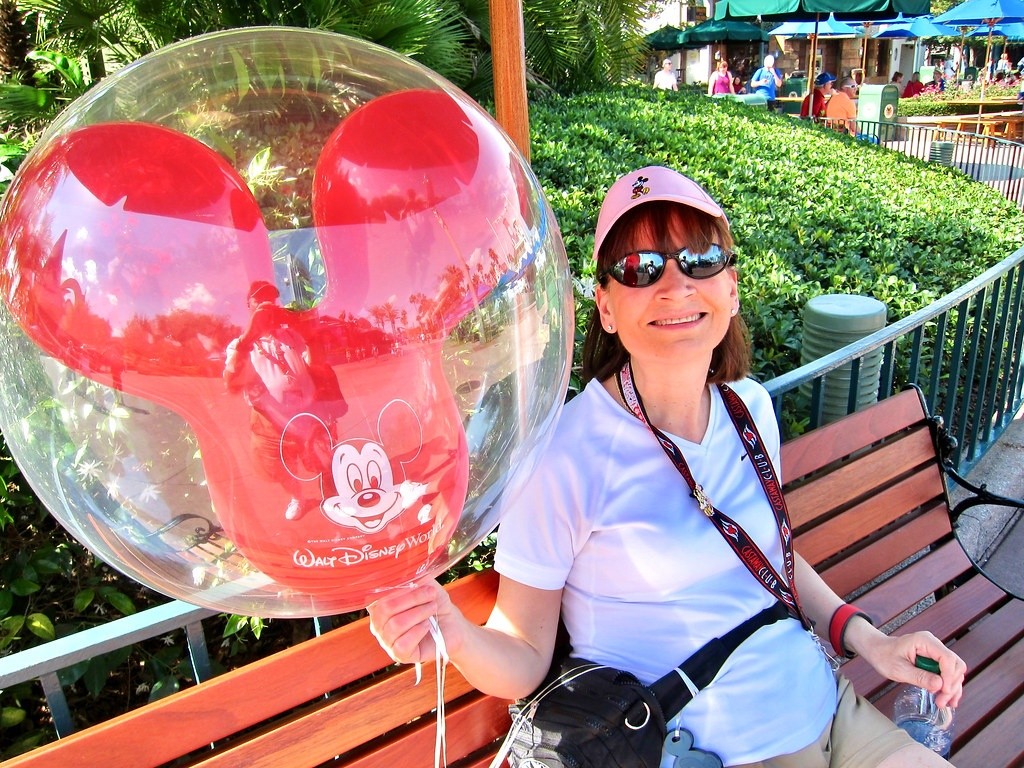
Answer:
[996,53,1009,71]
[751,55,784,111]
[647,260,657,283]
[890,71,904,97]
[223,280,348,518]
[1017,56,1024,74]
[901,71,924,98]
[681,256,694,275]
[708,61,735,96]
[366,168,968,768]
[732,77,747,94]
[652,59,679,91]
[826,76,858,132]
[932,67,946,91]
[801,72,836,122]
[979,56,1022,89]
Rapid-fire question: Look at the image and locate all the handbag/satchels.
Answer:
[504,657,667,768]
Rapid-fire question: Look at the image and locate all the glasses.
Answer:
[602,241,736,287]
[844,84,858,89]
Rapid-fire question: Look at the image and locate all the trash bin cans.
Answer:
[919,66,935,85]
[964,67,976,82]
[857,85,898,141]
[783,78,808,114]
[712,93,767,109]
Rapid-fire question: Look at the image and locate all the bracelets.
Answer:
[829,603,874,660]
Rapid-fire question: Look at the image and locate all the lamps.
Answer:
[858,47,863,56]
[888,49,892,55]
[773,49,779,59]
[714,50,721,61]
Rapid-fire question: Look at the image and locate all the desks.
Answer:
[947,96,1024,105]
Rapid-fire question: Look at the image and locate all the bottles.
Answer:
[889,655,956,762]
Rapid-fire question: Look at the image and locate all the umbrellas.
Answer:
[932,0,1024,82]
[767,16,862,95]
[677,16,771,60]
[642,23,707,59]
[714,0,932,117]
[841,12,932,84]
[873,19,962,72]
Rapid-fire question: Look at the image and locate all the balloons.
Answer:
[0,24,571,621]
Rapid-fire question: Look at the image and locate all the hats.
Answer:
[815,72,837,85]
[594,166,730,261]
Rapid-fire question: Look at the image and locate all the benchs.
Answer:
[0,378,1024,768]
[935,116,1023,147]
